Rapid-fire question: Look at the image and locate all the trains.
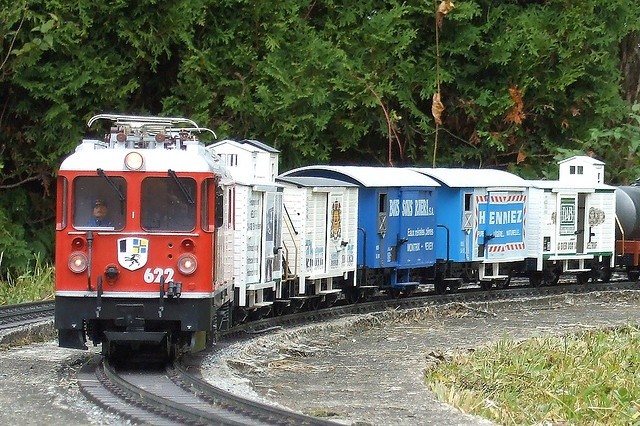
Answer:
[54,114,640,364]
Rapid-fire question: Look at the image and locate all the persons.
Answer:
[85,198,113,227]
